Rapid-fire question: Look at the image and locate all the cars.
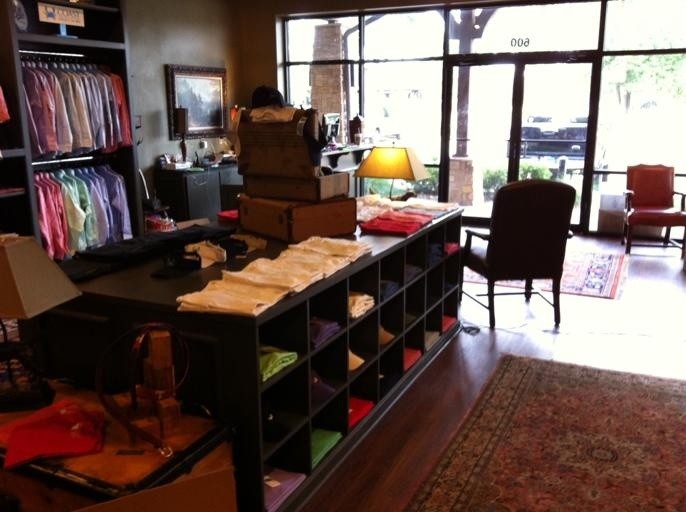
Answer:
[505,111,613,185]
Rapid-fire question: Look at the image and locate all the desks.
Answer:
[320,141,375,197]
[1,374,233,501]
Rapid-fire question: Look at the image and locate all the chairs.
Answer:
[460,181,575,330]
[620,164,686,258]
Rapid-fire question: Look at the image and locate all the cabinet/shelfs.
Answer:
[159,164,244,224]
[0,1,146,243]
[77,199,463,512]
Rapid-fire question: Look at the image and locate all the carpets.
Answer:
[401,354,686,510]
[462,246,626,299]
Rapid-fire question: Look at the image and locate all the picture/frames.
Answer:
[165,62,231,139]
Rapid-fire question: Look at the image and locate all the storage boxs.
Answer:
[239,105,359,242]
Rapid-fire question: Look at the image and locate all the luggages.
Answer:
[235,105,355,203]
[235,192,356,243]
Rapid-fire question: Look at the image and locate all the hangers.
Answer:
[35,160,110,174]
[17,52,103,71]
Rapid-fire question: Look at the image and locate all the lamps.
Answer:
[354,143,430,199]
[173,107,191,170]
[0,235,78,408]
